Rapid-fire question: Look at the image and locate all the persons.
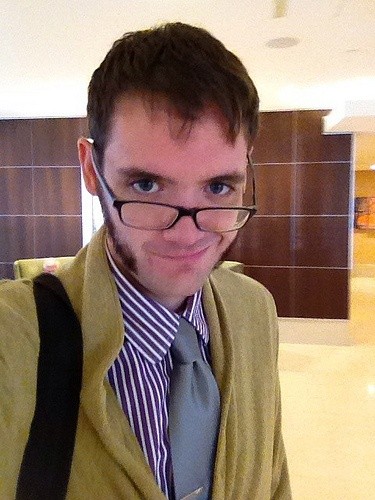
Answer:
[0,21,296,500]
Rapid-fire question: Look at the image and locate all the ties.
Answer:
[169,315,222,499]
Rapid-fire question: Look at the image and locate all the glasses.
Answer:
[87,138,257,233]
